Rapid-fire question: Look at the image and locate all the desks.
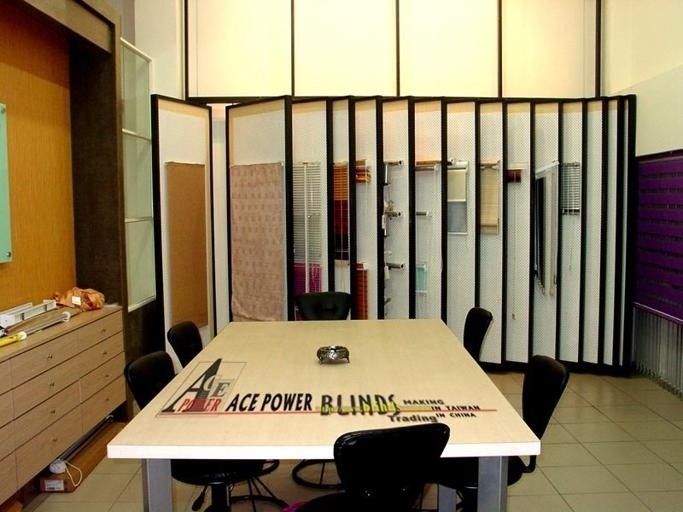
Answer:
[105,318,542,512]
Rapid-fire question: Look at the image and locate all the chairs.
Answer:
[292,422,453,512]
[124,350,292,512]
[165,319,204,368]
[461,306,494,362]
[432,354,572,511]
[292,292,353,321]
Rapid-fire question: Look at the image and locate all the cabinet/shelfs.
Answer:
[0,305,126,506]
